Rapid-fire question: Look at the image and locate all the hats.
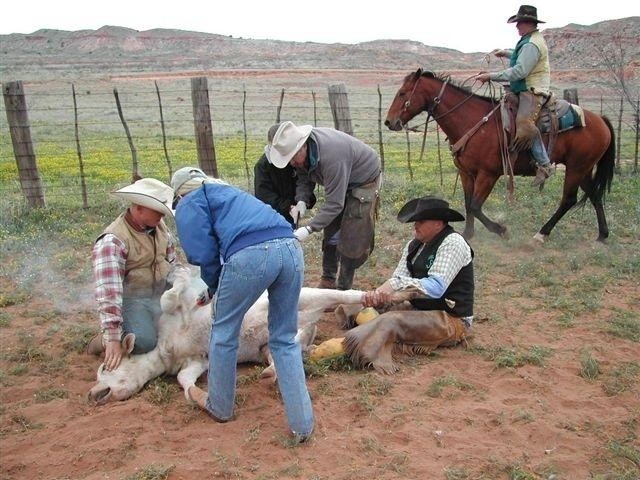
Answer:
[270,121,313,168]
[110,167,207,219]
[507,5,545,23]
[397,197,464,222]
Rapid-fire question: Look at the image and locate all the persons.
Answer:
[170,166,315,441]
[86,178,187,372]
[361,193,475,347]
[475,5,556,187]
[270,121,383,290]
[254,124,316,232]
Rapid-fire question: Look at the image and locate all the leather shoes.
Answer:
[188,386,227,422]
[531,163,554,186]
[87,333,105,355]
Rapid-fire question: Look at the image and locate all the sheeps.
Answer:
[86,285,433,405]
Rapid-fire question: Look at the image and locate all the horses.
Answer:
[385,67,617,248]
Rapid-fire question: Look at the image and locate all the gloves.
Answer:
[290,201,306,223]
[293,225,312,241]
